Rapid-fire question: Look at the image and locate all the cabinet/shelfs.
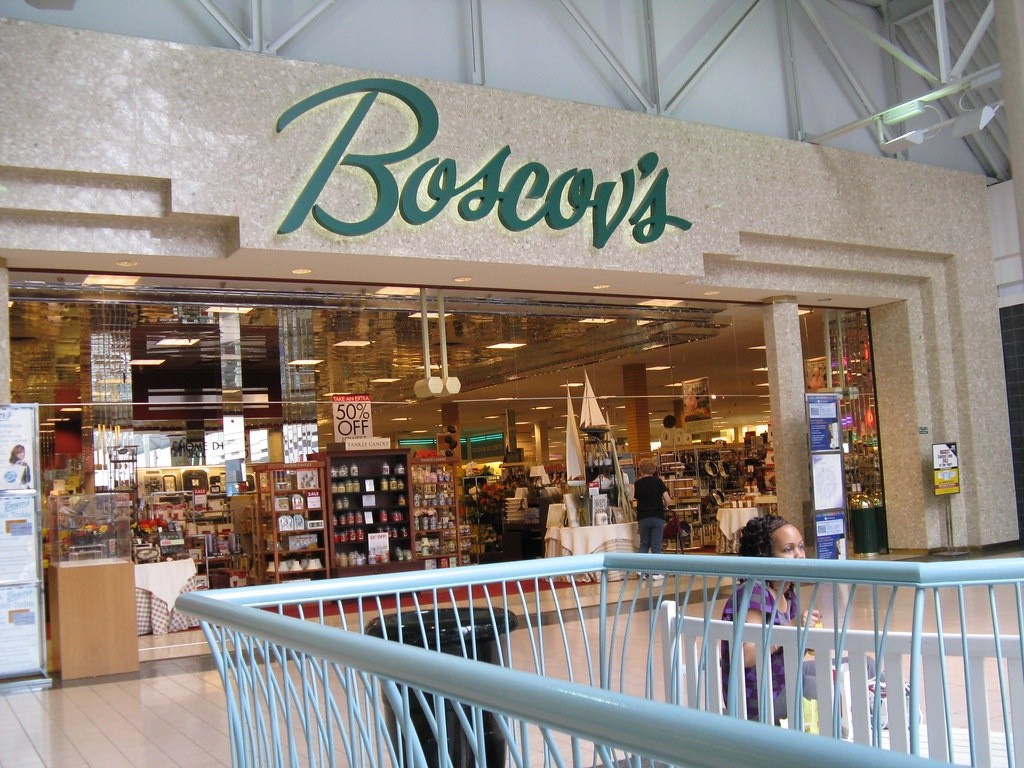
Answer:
[843,451,882,534]
[42,440,779,587]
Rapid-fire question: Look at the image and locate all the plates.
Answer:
[504,497,525,524]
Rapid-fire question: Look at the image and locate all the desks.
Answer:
[715,507,770,554]
[134,557,203,636]
[543,522,641,583]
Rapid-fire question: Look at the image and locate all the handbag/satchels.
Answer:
[0,459,28,488]
[663,508,682,540]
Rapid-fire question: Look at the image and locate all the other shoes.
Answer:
[636,571,646,580]
[652,574,664,581]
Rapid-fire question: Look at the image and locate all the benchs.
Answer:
[656,596,1024,768]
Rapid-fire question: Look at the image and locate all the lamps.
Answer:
[950,83,1006,141]
[882,101,943,154]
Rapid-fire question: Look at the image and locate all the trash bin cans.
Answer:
[364,604,517,768]
[871,496,889,553]
[850,496,880,557]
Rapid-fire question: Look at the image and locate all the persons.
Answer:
[9,445,31,489]
[592,466,629,489]
[723,516,887,722]
[633,458,671,580]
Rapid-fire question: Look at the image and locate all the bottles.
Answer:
[398,494,406,506]
[379,508,404,524]
[333,495,350,510]
[331,478,405,494]
[394,546,412,561]
[376,525,408,538]
[335,549,368,568]
[330,462,357,479]
[334,527,365,543]
[808,611,824,657]
[732,478,765,508]
[368,548,390,565]
[380,460,405,476]
[333,510,363,526]
